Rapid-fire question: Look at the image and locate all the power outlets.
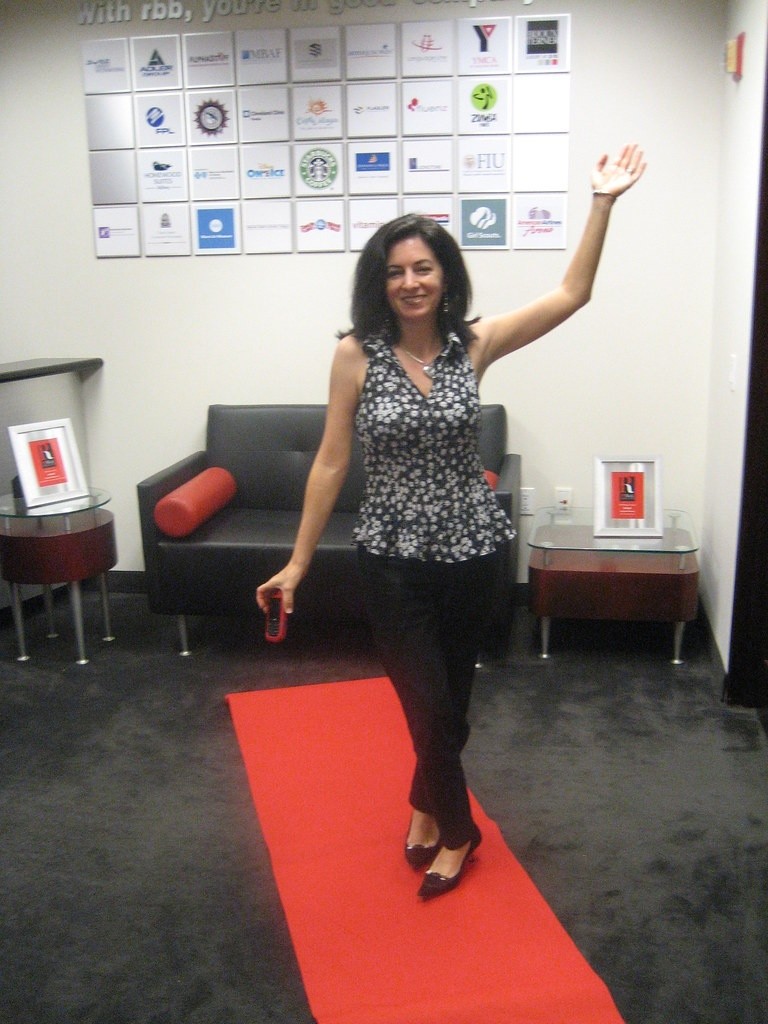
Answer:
[519,487,535,517]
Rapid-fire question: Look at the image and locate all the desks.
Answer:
[0,487,118,665]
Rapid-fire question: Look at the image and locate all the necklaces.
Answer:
[396,342,435,379]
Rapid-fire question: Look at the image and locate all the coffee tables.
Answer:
[526,523,700,666]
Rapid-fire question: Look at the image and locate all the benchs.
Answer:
[137,403,522,660]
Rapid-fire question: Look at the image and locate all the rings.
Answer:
[626,169,633,174]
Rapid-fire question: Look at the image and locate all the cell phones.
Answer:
[264,591,291,643]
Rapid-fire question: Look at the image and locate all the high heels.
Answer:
[405,809,442,871]
[417,824,481,897]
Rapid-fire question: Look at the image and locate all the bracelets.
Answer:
[592,190,617,198]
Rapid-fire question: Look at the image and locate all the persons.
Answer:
[257,141,646,896]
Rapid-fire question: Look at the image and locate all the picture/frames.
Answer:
[8,417,91,509]
[593,453,663,538]
